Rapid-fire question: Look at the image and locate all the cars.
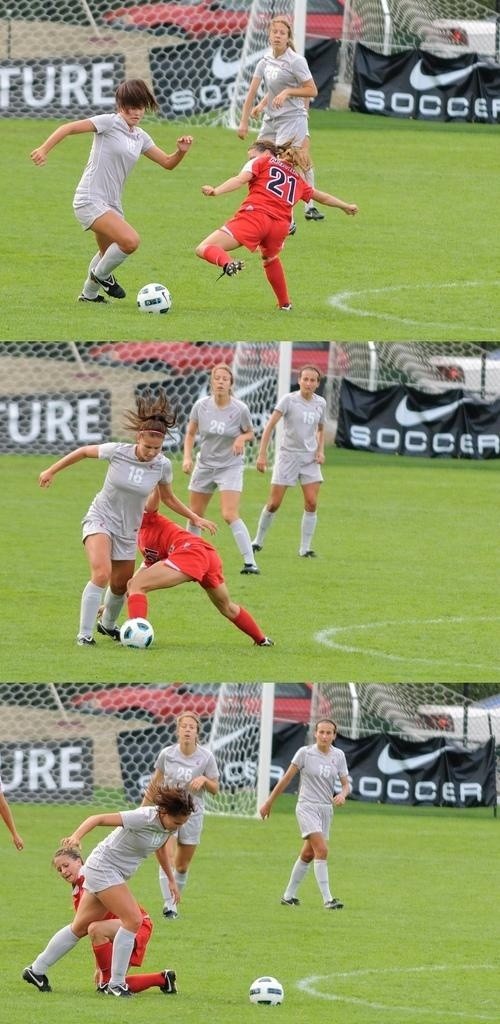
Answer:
[107,0,359,42]
[88,343,346,382]
[78,685,331,736]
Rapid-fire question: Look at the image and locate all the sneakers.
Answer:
[91,266,126,298]
[281,898,300,906]
[282,303,292,312]
[304,551,317,560]
[252,544,263,553]
[162,905,178,919]
[305,208,325,220]
[97,620,122,640]
[79,293,109,302]
[223,259,245,275]
[324,899,343,908]
[258,637,274,646]
[98,981,135,997]
[77,635,97,646]
[241,563,260,574]
[160,970,178,994]
[22,965,52,991]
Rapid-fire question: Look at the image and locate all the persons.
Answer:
[197,140,358,310]
[0,784,24,850]
[138,710,221,916]
[181,364,266,574]
[51,847,179,996]
[22,784,195,996]
[260,719,351,912]
[96,480,275,648]
[39,396,217,648]
[30,79,193,304]
[254,366,330,560]
[237,16,326,236]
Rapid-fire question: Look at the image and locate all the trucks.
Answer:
[430,357,500,397]
[434,17,495,57]
[416,706,500,748]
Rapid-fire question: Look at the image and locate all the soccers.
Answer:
[249,977,285,1006]
[119,618,155,648]
[136,283,172,314]
